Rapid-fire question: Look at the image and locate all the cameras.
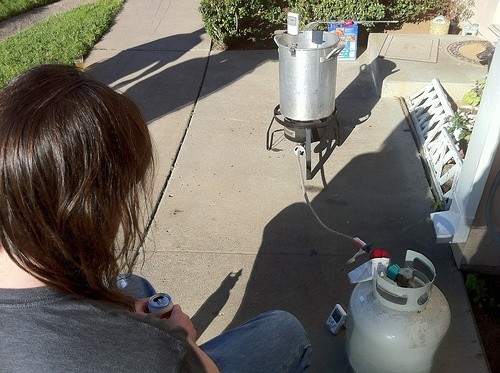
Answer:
[325,304,347,334]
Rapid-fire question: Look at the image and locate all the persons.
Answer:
[0,61,314,372]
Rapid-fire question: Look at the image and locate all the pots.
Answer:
[273,31,346,121]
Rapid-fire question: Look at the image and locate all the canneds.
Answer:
[147,293,173,319]
